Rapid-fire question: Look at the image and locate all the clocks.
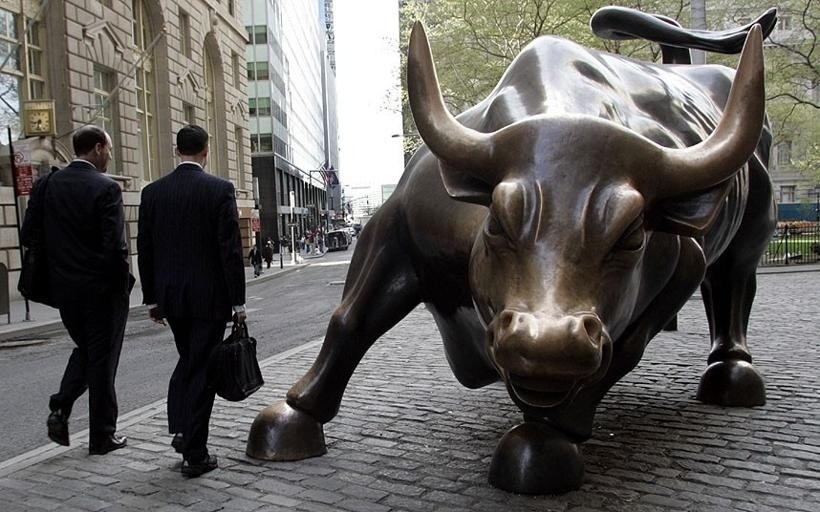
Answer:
[23,99,56,137]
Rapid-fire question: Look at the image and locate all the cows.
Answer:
[242,1,782,496]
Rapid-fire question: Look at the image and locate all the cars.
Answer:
[325,221,361,251]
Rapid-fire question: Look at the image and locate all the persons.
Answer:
[262,223,333,270]
[137,125,248,479]
[17,124,135,455]
[248,244,262,278]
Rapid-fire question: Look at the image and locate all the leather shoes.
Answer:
[89,436,127,455]
[171,433,218,476]
[47,409,69,446]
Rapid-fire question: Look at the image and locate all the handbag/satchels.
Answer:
[217,320,264,401]
